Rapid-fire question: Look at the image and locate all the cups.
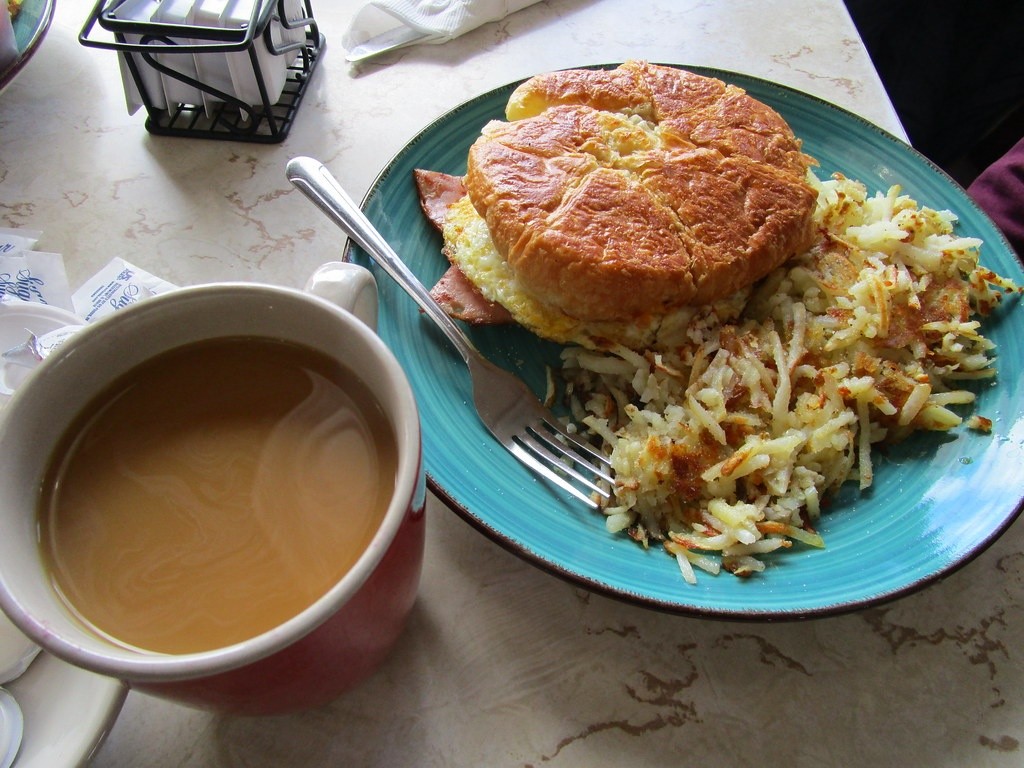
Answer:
[0,258,430,719]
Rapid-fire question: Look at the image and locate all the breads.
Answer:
[466,61,817,322]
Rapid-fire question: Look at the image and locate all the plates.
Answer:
[0,303,133,768]
[338,59,1024,620]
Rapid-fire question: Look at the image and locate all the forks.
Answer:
[282,154,617,511]
